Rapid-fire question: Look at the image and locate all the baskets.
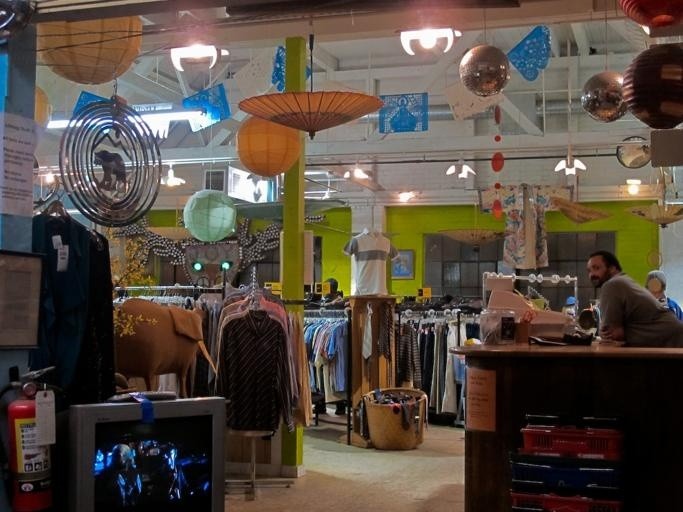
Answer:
[366,388,426,450]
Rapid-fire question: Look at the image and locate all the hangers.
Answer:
[247,265,264,324]
[304,310,348,318]
[116,285,210,301]
[400,310,466,319]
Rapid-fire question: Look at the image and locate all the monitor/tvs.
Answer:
[225,164,276,204]
[51,397,226,512]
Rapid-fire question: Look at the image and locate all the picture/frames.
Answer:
[391,248,415,280]
[0,248,49,351]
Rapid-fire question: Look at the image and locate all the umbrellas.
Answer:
[235,29,388,140]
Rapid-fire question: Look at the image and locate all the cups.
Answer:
[479,310,501,345]
[514,322,529,346]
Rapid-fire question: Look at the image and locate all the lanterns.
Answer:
[617,1,683,29]
[33,88,55,130]
[235,117,307,179]
[620,41,683,127]
[36,15,145,85]
[180,185,237,246]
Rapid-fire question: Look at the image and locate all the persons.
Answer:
[320,278,345,310]
[584,249,682,348]
[643,268,682,321]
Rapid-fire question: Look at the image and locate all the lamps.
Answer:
[399,22,464,59]
[342,162,374,181]
[444,161,479,181]
[553,157,588,178]
[156,163,188,188]
[168,40,220,75]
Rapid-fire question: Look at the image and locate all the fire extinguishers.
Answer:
[0,365,63,512]
